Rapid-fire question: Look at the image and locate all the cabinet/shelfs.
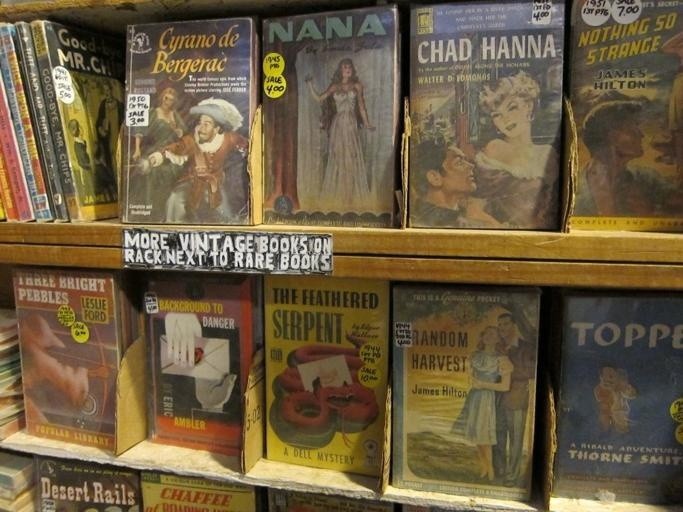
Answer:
[0,0,682,512]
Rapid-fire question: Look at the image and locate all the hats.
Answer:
[187,103,226,124]
[155,79,183,96]
